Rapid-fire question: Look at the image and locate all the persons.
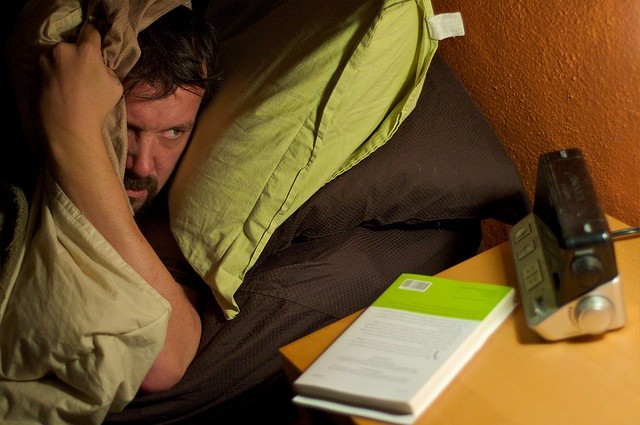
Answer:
[24,4,225,395]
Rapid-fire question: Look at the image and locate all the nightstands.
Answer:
[278,213,640,425]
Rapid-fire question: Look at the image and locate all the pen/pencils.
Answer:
[558,226,640,249]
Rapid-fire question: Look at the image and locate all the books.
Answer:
[287,268,519,425]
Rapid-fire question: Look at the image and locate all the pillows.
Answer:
[253,53,536,271]
[165,0,440,321]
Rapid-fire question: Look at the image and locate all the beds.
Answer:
[0,0,534,425]
